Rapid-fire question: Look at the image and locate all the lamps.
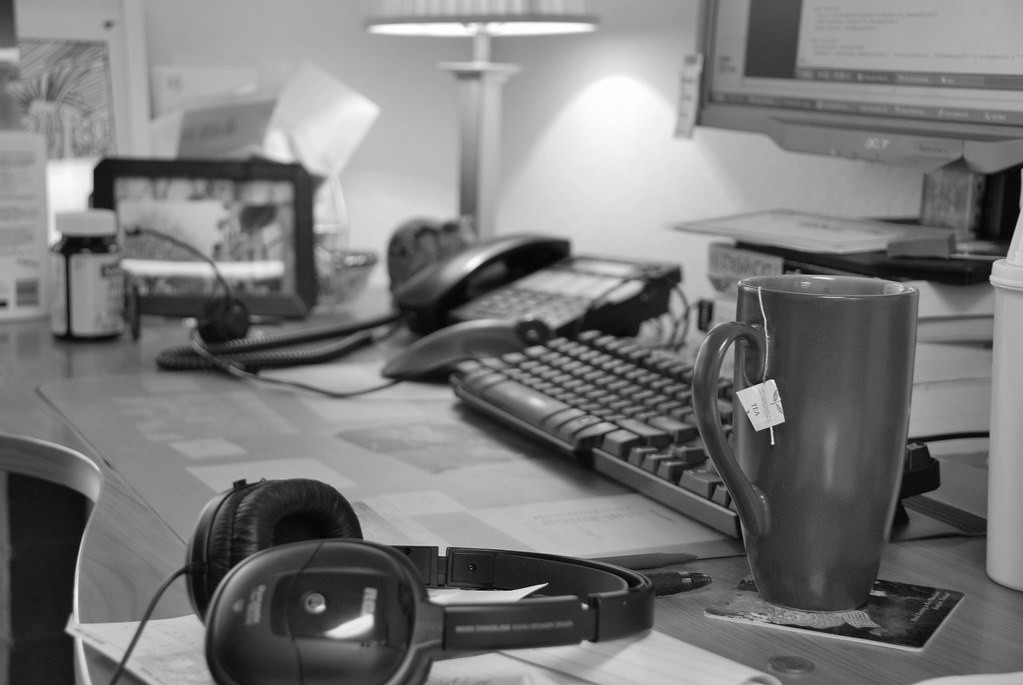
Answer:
[363,0,599,247]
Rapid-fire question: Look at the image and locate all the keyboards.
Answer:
[450,332,941,539]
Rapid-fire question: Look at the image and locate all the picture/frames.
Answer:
[93,157,318,321]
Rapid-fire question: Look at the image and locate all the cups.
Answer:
[692,274,920,613]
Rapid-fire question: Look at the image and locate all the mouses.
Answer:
[381,313,553,382]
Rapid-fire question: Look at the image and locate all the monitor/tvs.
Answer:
[692,0,1023,284]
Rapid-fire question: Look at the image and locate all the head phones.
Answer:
[186,477,654,685]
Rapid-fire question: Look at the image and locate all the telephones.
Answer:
[393,231,683,342]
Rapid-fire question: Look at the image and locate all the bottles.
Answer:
[50,209,124,343]
[986,171,1023,591]
